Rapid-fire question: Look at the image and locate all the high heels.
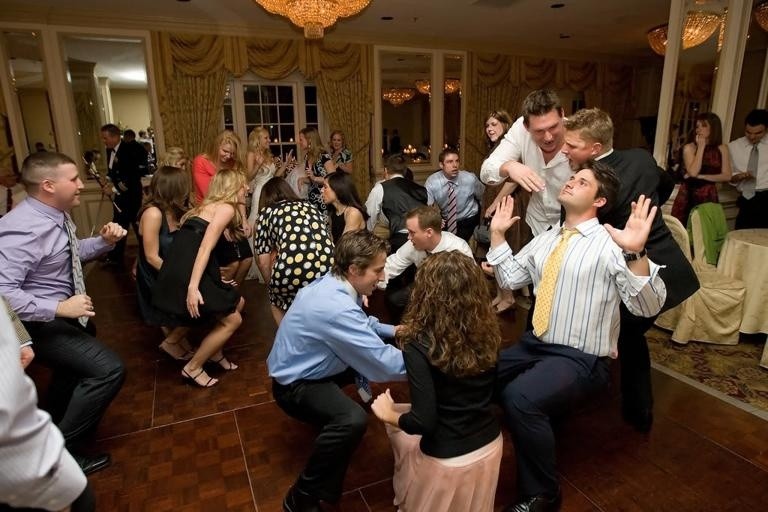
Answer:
[207,357,239,372]
[492,298,519,316]
[180,366,219,388]
[156,339,197,363]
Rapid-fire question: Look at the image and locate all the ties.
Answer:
[446,182,457,235]
[531,227,580,339]
[64,220,91,329]
[741,142,760,201]
[354,371,374,404]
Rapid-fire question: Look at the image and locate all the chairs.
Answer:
[691,201,727,271]
[653,213,747,346]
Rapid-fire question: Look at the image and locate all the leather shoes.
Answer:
[620,401,655,432]
[500,489,564,512]
[280,484,326,512]
[69,452,111,477]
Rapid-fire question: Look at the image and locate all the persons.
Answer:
[1,304,88,512]
[1,295,35,370]
[0,156,28,218]
[0,152,129,476]
[34,141,47,152]
[727,108,768,230]
[84,89,731,512]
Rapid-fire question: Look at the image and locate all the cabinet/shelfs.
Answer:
[81,189,153,247]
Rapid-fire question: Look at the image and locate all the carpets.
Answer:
[646,325,768,420]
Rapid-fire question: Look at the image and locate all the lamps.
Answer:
[254,1,375,40]
[645,4,721,58]
[382,88,414,108]
[416,79,462,100]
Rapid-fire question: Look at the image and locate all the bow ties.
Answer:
[109,148,116,154]
